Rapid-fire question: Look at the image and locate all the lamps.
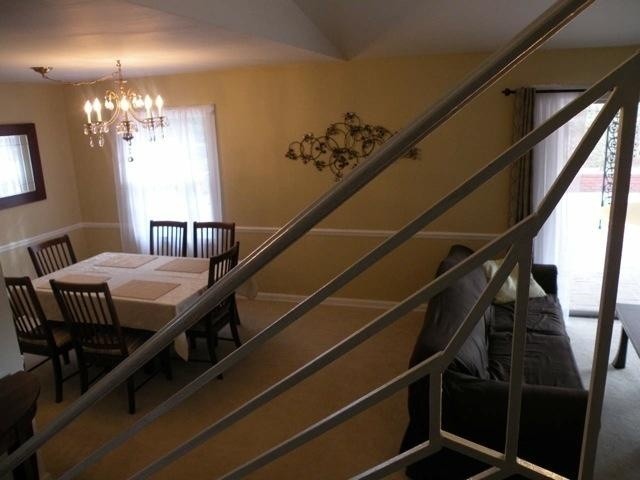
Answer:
[81,60,168,166]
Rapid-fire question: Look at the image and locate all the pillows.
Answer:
[482,256,547,304]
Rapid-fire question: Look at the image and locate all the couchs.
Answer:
[404,237,588,474]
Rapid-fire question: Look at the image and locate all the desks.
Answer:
[610,297,640,365]
